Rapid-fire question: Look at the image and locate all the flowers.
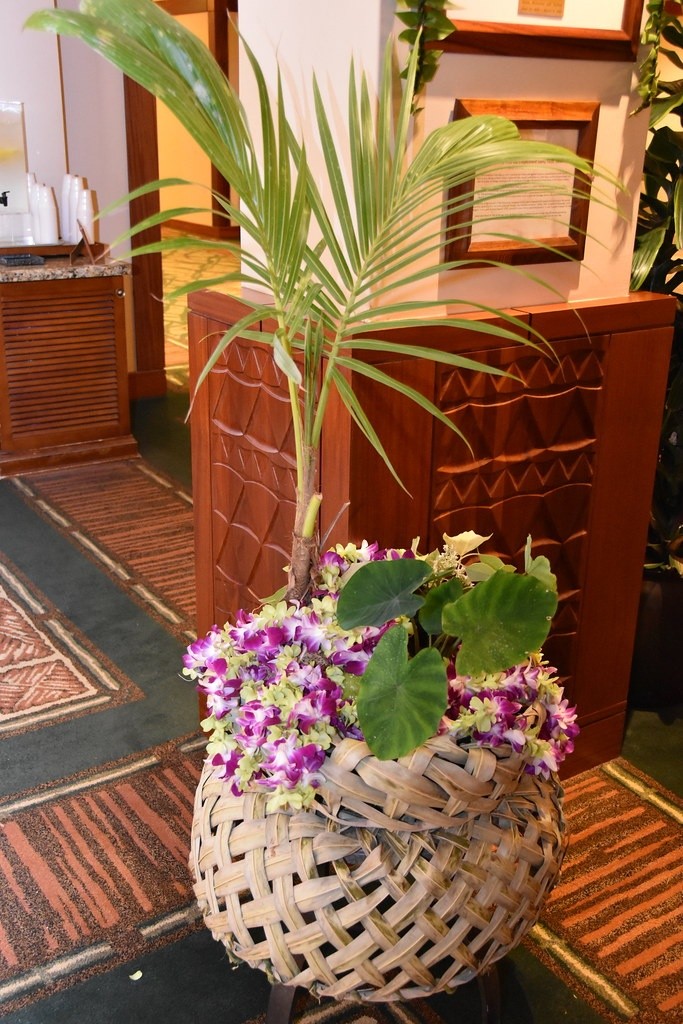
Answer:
[175,525,580,821]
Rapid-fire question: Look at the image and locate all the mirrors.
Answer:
[0,1,68,253]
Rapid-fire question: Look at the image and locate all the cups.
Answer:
[59,174,95,244]
[27,173,58,245]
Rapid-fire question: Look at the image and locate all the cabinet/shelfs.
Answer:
[0,276,142,478]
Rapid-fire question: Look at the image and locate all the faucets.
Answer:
[0,190,10,206]
